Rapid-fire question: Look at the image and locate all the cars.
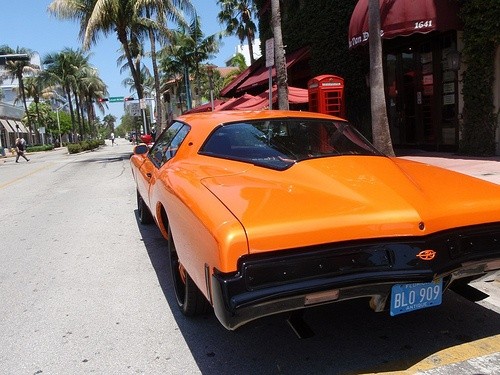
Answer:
[129,110,499,332]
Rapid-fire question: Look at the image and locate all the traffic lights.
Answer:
[125,98,134,101]
[99,99,108,101]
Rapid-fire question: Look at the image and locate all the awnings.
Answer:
[219,46,312,97]
[348,0,465,48]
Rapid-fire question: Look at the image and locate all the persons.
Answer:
[47,136,59,144]
[131,129,156,146]
[109,130,118,147]
[15,136,30,163]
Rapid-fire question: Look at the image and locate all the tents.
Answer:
[183,84,308,115]
[0,119,34,133]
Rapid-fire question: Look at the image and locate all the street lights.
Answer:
[57,101,69,148]
